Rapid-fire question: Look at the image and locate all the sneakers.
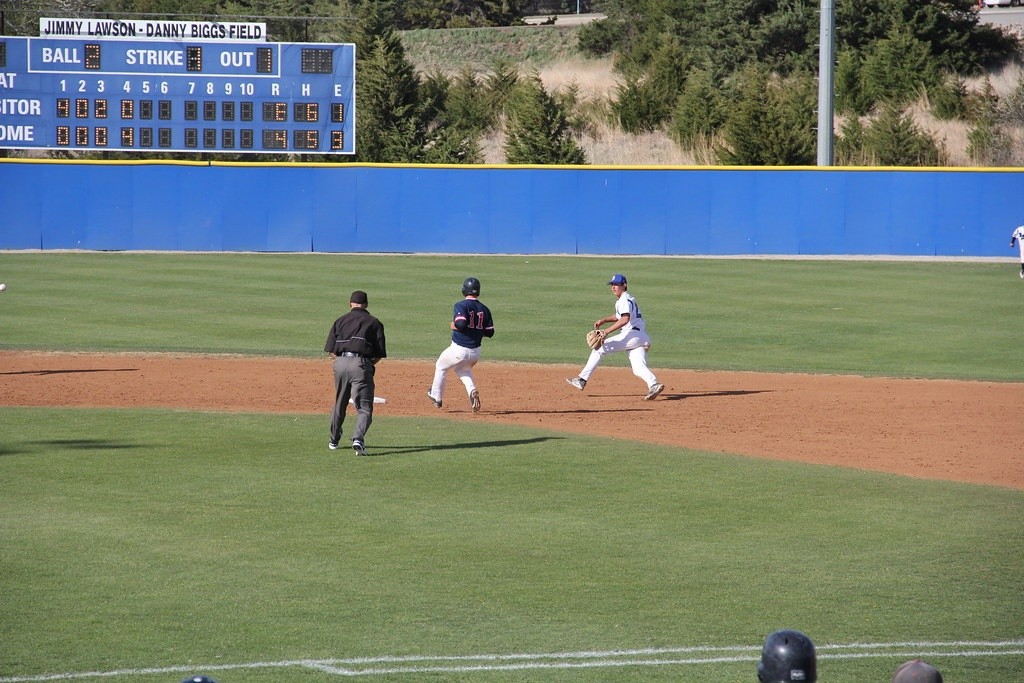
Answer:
[427,388,442,408]
[644,383,665,399]
[566,377,586,390]
[351,440,368,456]
[329,442,339,449]
[469,389,481,412]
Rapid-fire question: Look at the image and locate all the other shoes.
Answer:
[1019,271,1024,278]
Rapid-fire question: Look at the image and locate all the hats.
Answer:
[888,659,944,683]
[350,291,368,303]
[607,274,627,284]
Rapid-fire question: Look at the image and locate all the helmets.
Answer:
[756,631,817,683]
[462,277,480,297]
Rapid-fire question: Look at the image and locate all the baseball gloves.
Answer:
[586,329,608,351]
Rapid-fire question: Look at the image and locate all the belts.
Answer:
[337,351,364,357]
[630,326,640,331]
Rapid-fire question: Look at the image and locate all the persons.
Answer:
[893,659,943,683]
[566,274,665,400]
[758,629,817,683]
[324,291,387,455]
[428,277,495,413]
[1010,226,1024,278]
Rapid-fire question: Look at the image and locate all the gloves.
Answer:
[1010,243,1014,247]
